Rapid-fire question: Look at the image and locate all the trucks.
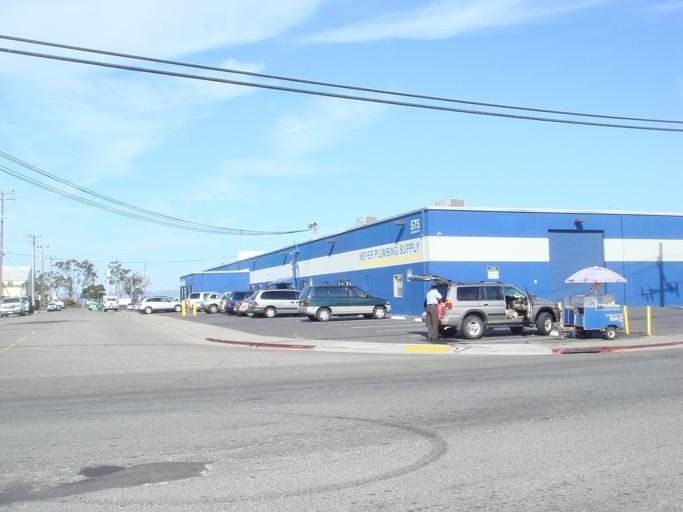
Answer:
[102,295,119,312]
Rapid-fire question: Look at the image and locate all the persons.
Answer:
[425,285,442,340]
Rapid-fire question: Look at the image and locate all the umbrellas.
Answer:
[564,265,627,295]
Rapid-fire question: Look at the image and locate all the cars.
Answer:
[0,295,64,318]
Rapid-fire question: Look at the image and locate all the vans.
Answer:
[187,291,261,317]
[247,288,301,318]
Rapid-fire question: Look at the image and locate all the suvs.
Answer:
[297,283,392,323]
[408,273,562,340]
[137,296,184,315]
